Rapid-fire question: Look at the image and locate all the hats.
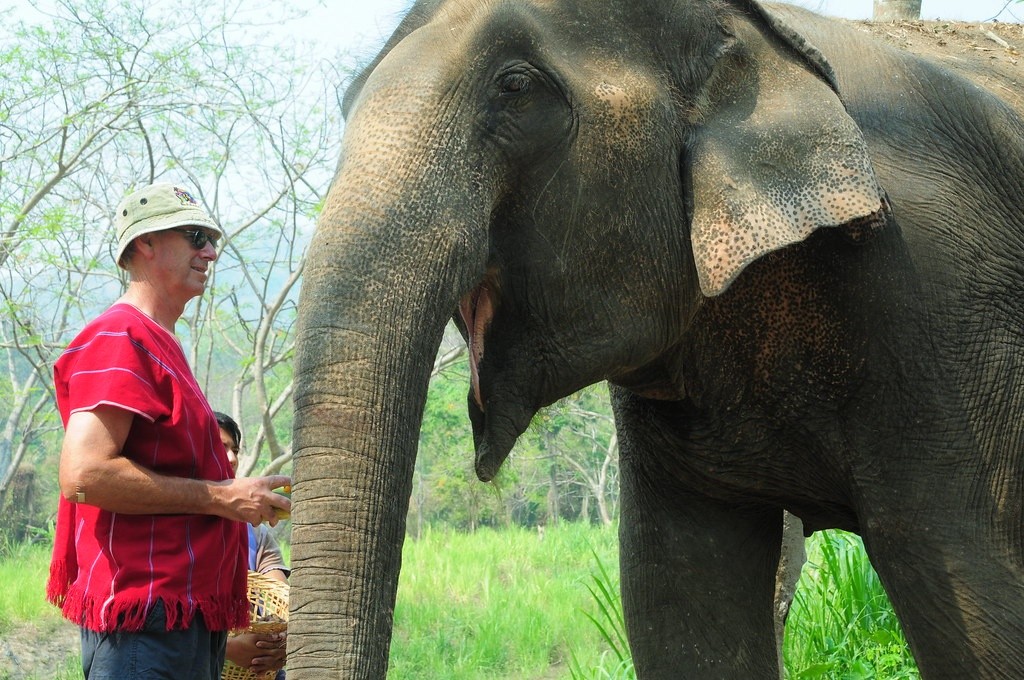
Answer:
[116,183,222,270]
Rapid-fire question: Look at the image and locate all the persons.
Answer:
[46,183,291,680]
[212,411,291,680]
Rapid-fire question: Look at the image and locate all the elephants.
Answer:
[283,0,1024,680]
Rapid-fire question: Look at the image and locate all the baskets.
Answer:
[220,569,290,680]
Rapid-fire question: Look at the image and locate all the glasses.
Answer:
[171,227,217,249]
